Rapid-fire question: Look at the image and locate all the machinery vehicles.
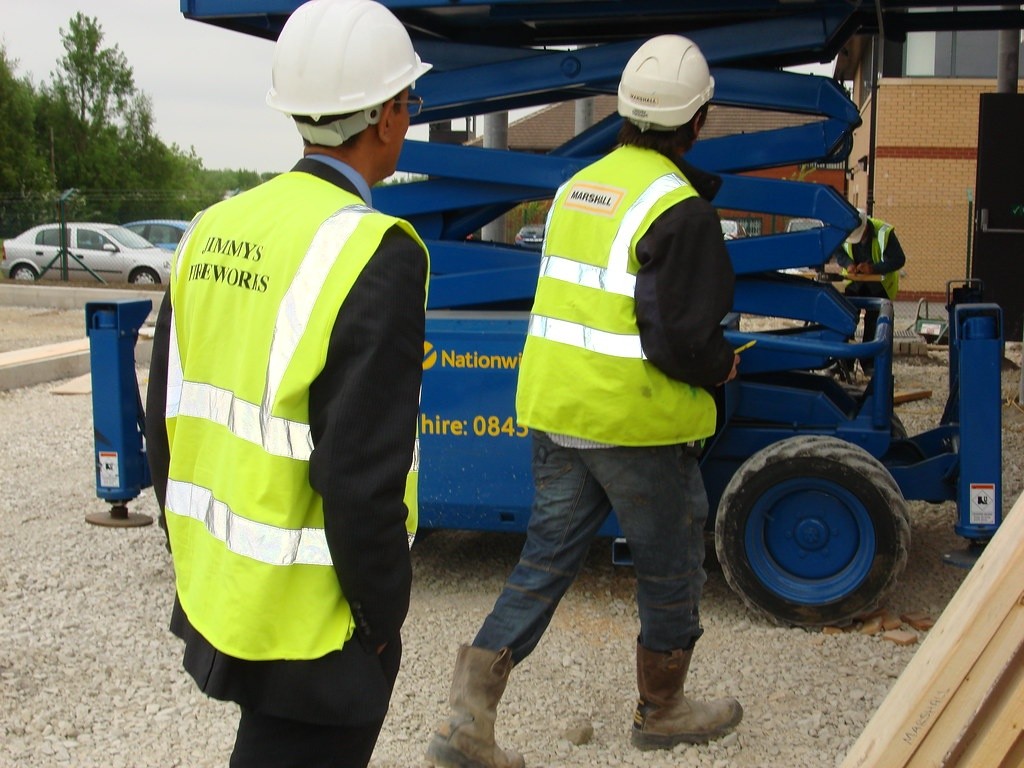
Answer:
[83,0,1007,633]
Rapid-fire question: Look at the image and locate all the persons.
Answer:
[420,35,743,768]
[145,0,431,768]
[828,207,906,378]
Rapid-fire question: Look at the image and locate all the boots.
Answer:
[630,633,743,752]
[425,643,525,768]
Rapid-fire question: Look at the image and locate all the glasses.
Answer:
[383,95,423,117]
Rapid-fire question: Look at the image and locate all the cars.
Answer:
[124,219,192,251]
[2,221,172,285]
[514,224,544,250]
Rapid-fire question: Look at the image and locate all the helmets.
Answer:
[844,208,867,244]
[618,35,715,133]
[272,0,433,122]
